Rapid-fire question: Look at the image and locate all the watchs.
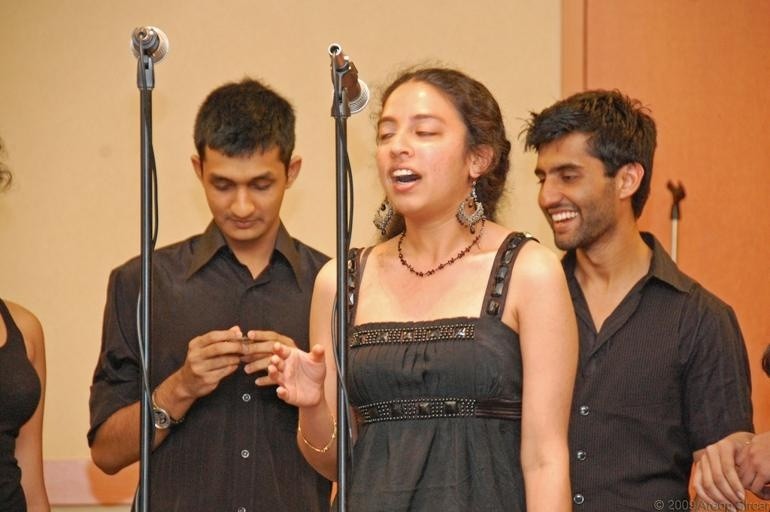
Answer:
[151,394,185,431]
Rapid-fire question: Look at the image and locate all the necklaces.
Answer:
[395,215,487,277]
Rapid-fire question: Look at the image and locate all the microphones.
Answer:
[328,43,370,116]
[129,23,169,63]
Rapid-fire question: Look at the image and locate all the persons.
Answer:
[0,143,52,512]
[267,68,580,511]
[518,88,754,511]
[691,341,770,512]
[86,79,332,512]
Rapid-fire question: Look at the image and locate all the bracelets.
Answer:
[298,413,337,453]
